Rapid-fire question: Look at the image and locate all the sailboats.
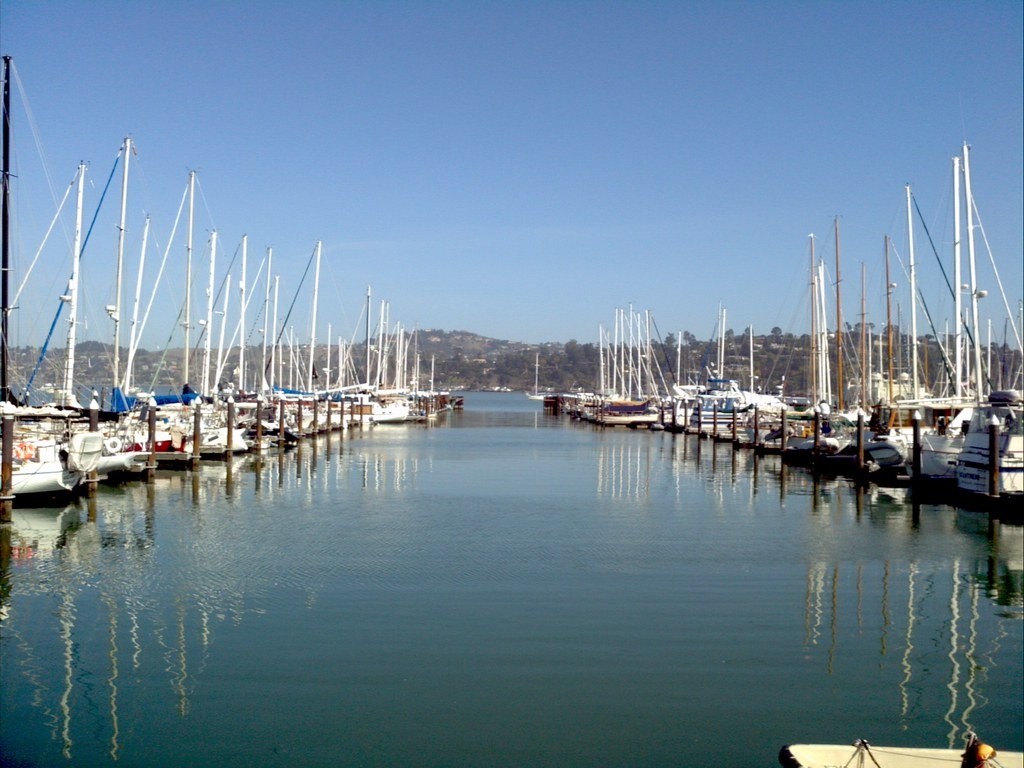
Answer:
[1,55,456,507]
[560,140,1024,518]
